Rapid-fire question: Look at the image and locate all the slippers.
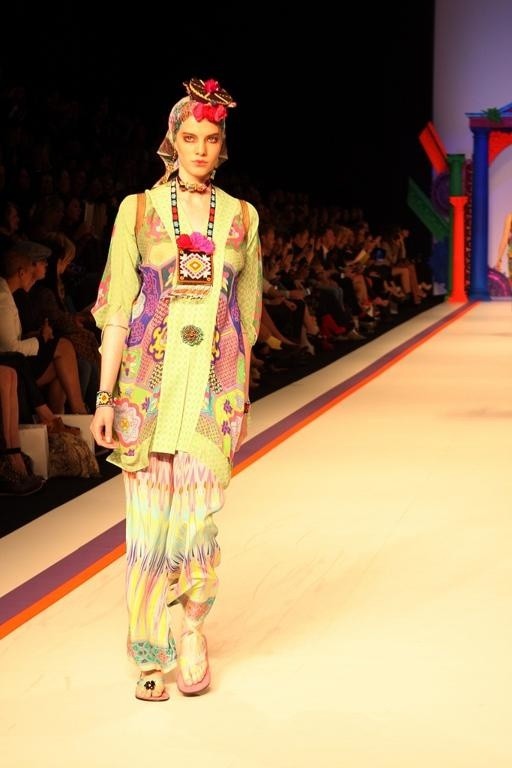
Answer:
[135,672,170,702]
[173,628,210,696]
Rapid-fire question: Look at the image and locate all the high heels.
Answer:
[322,319,346,337]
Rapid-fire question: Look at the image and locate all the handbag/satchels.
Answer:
[19,415,101,481]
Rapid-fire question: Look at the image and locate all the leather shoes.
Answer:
[269,342,308,356]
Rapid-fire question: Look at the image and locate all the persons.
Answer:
[1,90,152,495]
[495,212,512,288]
[217,169,432,389]
[89,95,264,702]
